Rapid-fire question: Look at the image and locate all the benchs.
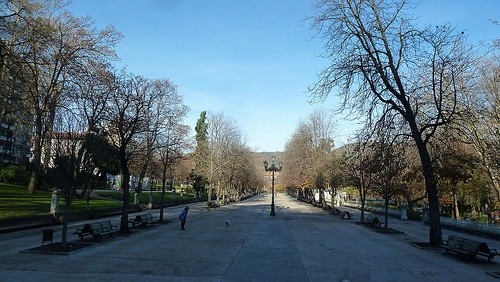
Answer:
[73,220,120,243]
[128,213,158,229]
[362,215,384,229]
[438,234,500,264]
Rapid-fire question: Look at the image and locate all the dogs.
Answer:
[223,219,233,232]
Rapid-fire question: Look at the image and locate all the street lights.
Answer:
[263,154,284,217]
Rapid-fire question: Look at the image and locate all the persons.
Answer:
[209,200,216,208]
[179,207,189,230]
[373,217,379,224]
